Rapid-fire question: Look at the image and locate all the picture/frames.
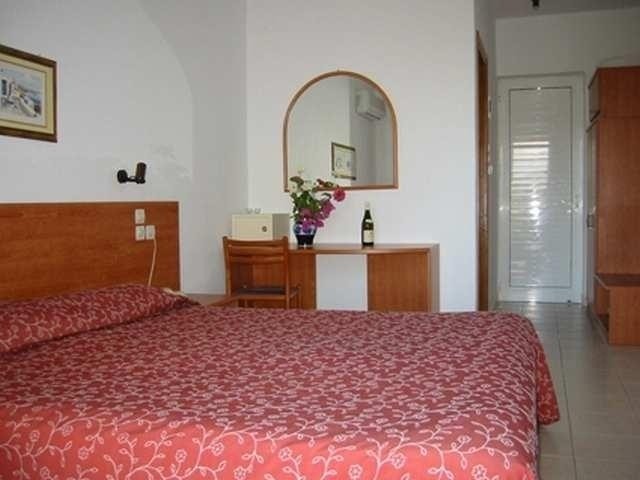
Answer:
[332,142,358,180]
[0,43,60,144]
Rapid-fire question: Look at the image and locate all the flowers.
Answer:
[287,176,345,231]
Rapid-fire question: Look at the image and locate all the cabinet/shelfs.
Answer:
[585,65,640,349]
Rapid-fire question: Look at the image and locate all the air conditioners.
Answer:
[356,87,386,122]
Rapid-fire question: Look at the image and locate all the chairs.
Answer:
[222,236,299,309]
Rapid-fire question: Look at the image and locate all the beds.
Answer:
[1,201,559,480]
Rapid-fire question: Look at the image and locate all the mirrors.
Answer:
[283,69,398,192]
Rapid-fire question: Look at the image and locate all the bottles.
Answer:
[361,203,374,246]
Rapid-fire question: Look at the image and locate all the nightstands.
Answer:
[187,293,240,308]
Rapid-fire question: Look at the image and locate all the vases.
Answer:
[293,219,317,248]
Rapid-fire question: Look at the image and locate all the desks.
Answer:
[227,243,440,313]
